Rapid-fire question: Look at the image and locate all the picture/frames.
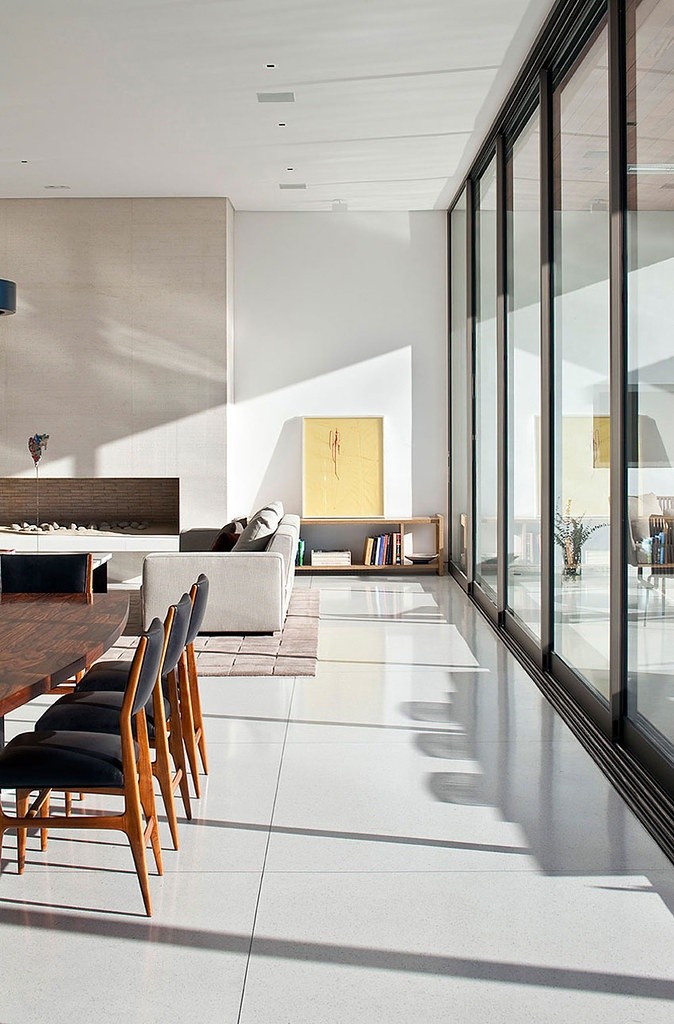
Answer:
[536,415,641,518]
[301,414,386,519]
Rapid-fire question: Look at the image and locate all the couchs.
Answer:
[140,515,301,632]
[609,494,674,567]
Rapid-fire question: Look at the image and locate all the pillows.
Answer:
[209,501,285,552]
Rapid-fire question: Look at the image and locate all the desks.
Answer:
[459,513,610,574]
[295,514,446,576]
[0,594,130,749]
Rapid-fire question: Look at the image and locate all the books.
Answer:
[365,533,401,565]
[300,540,304,566]
[651,532,674,564]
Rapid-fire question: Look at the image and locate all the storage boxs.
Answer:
[310,549,351,567]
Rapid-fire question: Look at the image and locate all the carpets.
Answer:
[88,587,321,678]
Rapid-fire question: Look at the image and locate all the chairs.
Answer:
[0,573,210,919]
[637,496,674,574]
[644,516,674,572]
[1,553,93,594]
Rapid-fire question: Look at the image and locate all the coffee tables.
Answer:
[1,553,112,594]
